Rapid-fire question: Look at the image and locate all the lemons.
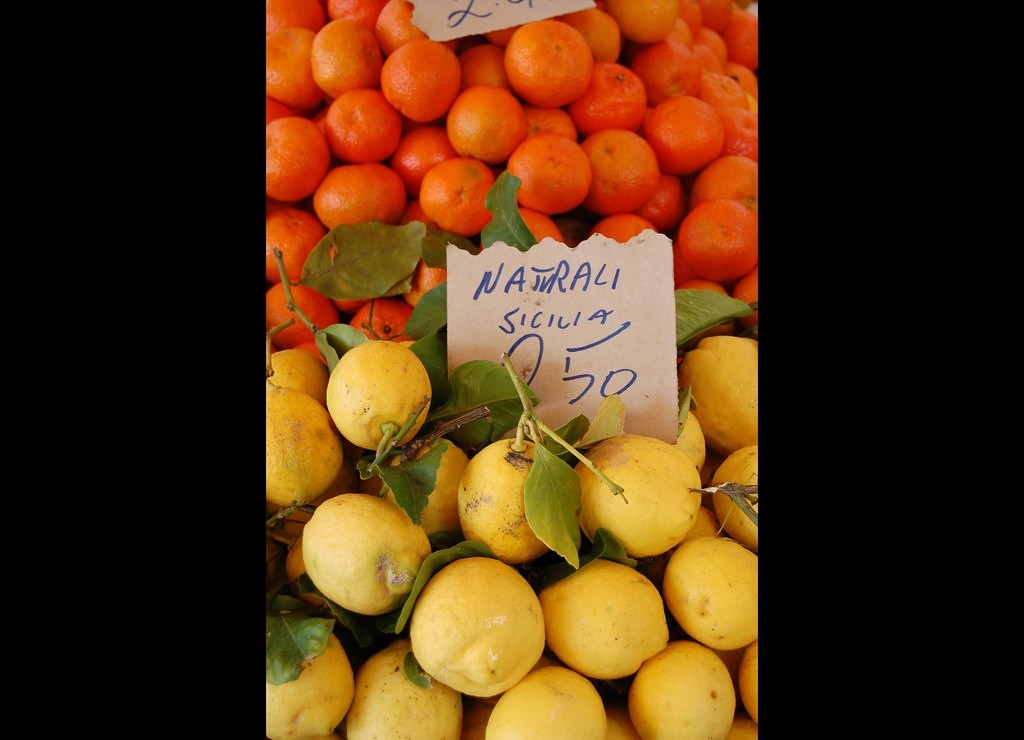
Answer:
[267,333,758,740]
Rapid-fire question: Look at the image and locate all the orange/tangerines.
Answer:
[266,0,758,359]
